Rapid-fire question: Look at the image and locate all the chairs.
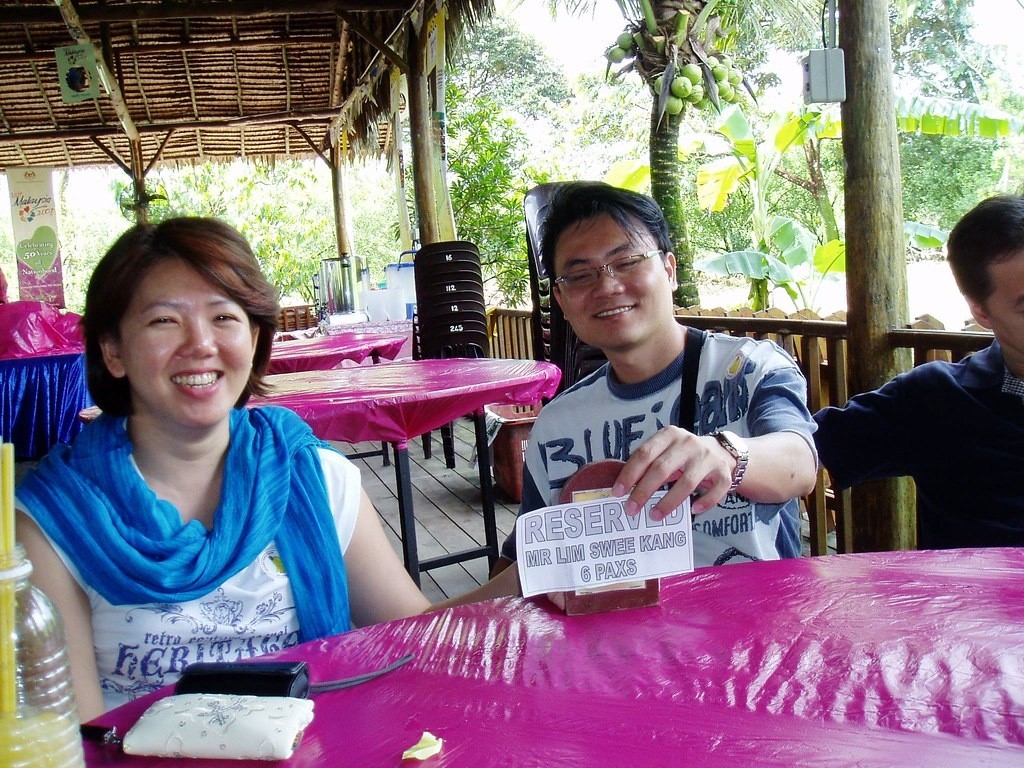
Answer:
[524,179,610,408]
[413,240,490,468]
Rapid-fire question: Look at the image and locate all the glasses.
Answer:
[554,250,665,291]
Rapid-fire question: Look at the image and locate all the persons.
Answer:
[13,216,522,724]
[431,181,819,608]
[813,196,1024,551]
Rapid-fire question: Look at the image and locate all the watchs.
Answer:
[703,430,750,494]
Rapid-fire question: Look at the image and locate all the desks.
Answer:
[83,547,1024,768]
[264,331,409,467]
[78,358,561,593]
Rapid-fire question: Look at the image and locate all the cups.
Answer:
[363,290,387,322]
[384,289,406,322]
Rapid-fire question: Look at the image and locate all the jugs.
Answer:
[384,250,417,320]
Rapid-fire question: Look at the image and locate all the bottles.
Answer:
[0,542,86,768]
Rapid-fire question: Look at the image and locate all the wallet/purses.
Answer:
[174,652,417,702]
[123,693,315,760]
